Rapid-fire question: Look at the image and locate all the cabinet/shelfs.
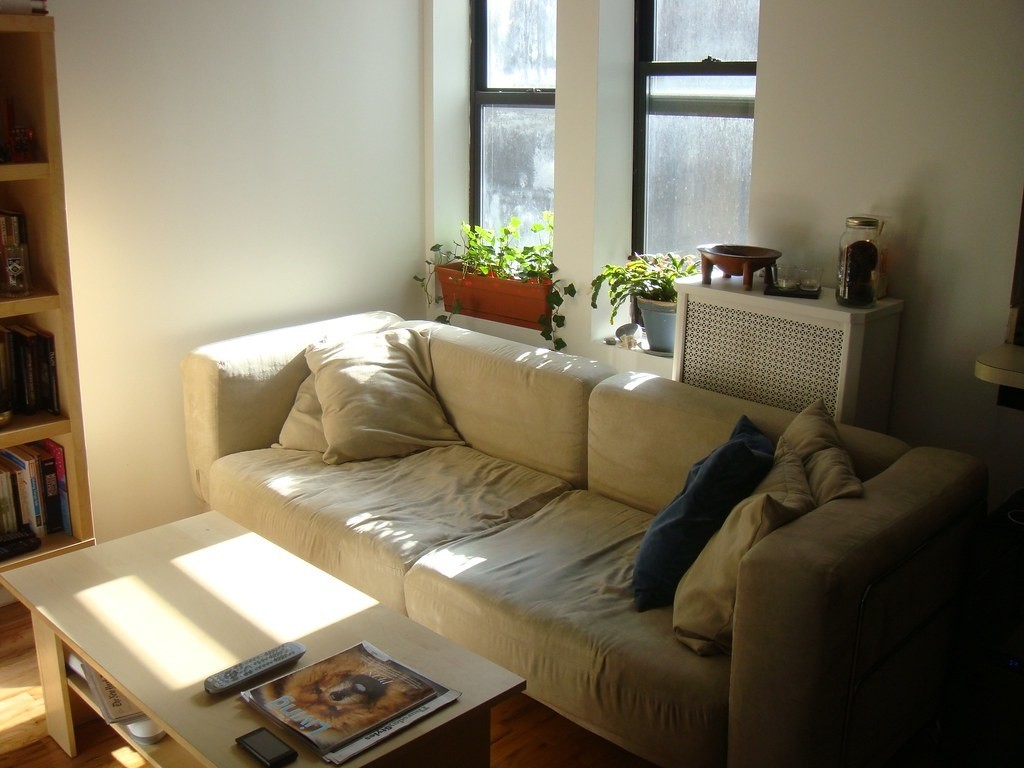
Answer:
[0,0,95,660]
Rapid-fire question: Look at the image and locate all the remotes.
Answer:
[205,642,307,695]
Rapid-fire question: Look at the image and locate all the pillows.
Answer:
[270,372,329,453]
[673,436,814,655]
[305,327,464,466]
[780,395,863,506]
[632,413,773,611]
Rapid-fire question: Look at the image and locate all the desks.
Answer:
[0,510,527,768]
[672,275,905,425]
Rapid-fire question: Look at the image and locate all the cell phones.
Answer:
[236,727,298,768]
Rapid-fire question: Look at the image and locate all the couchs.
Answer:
[182,312,1003,768]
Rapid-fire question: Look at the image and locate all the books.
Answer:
[0,438,72,537]
[249,643,436,756]
[0,325,60,427]
[0,208,28,248]
[80,660,145,724]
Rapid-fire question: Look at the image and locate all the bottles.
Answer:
[836,217,881,308]
[0,217,31,298]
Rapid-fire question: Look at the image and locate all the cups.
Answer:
[776,264,824,291]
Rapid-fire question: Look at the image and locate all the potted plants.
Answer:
[412,212,577,349]
[587,251,700,353]
